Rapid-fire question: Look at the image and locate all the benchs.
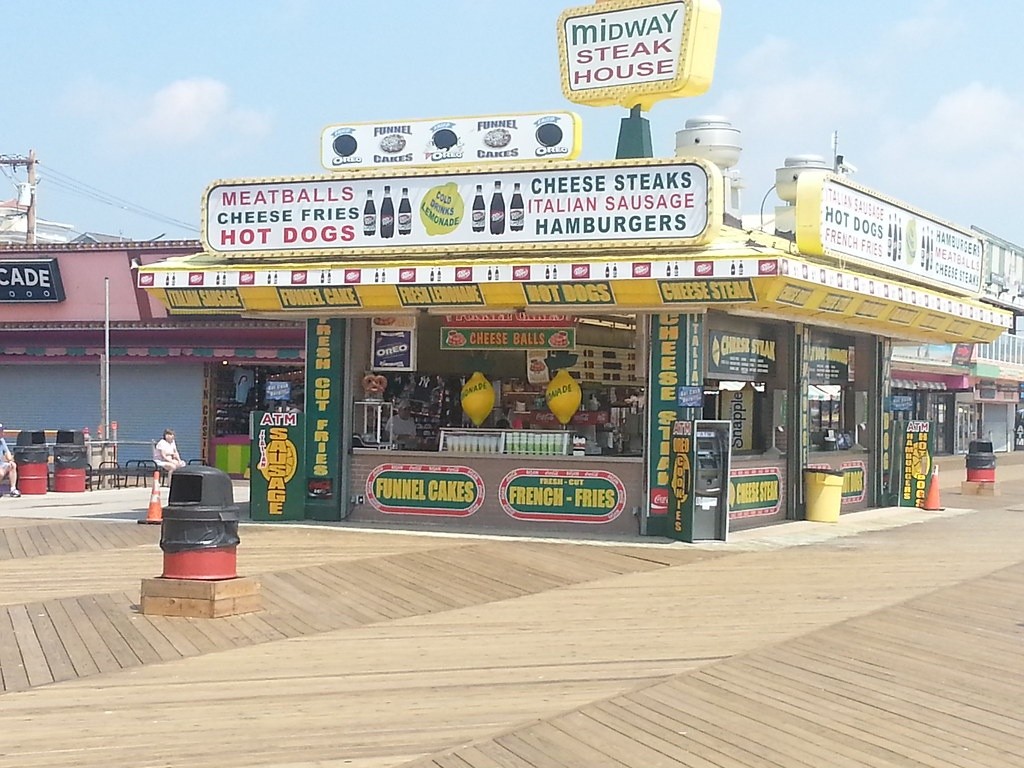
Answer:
[48,457,208,487]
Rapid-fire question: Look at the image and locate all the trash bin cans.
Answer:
[804,468,845,523]
[160,464,241,581]
[53,428,88,493]
[13,429,50,495]
[965,439,997,482]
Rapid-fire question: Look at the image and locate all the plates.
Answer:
[548,340,570,347]
[446,338,467,346]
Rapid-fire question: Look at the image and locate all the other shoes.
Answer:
[10,488,21,497]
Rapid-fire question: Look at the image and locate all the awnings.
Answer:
[890,377,947,391]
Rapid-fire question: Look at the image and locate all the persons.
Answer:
[153,428,186,486]
[0,422,21,497]
[385,400,416,437]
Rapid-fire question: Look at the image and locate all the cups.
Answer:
[502,384,511,392]
[506,432,563,456]
[516,401,525,411]
[447,435,501,453]
[530,358,546,381]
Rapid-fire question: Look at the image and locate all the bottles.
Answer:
[398,188,412,234]
[921,228,925,267]
[375,269,385,282]
[888,215,892,257]
[471,185,485,232]
[510,183,524,231]
[215,391,248,435]
[381,186,394,238]
[267,270,277,284]
[495,266,499,280]
[613,263,617,277]
[216,272,226,285]
[166,273,175,286]
[893,214,897,261]
[553,265,557,279]
[731,260,743,275]
[545,265,549,278]
[364,190,376,235]
[674,262,678,276]
[605,263,609,277]
[429,268,441,281]
[488,266,491,280]
[898,218,901,260]
[926,226,929,271]
[667,262,671,275]
[490,180,505,235]
[930,230,933,269]
[321,270,331,284]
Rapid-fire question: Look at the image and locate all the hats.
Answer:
[398,400,412,408]
[0,423,7,430]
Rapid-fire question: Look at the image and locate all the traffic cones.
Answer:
[922,463,949,512]
[136,471,164,524]
